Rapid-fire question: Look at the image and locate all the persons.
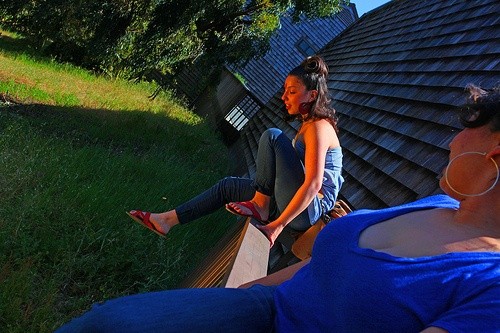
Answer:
[126,55,344,248]
[56,84,500,333]
